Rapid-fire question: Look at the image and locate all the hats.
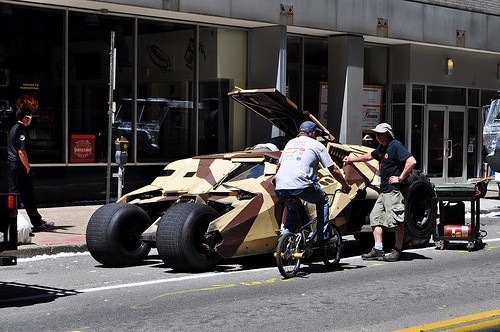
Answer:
[300,120,322,134]
[370,123,395,139]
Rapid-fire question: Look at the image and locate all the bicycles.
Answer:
[276,188,349,279]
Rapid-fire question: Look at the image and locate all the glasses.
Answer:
[25,115,33,120]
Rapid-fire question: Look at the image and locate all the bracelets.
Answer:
[398,176,400,183]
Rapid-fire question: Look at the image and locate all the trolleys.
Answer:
[434,180,489,252]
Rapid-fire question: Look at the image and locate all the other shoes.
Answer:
[318,232,338,245]
[278,230,295,244]
[32,222,54,232]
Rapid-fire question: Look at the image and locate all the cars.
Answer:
[86,85,440,273]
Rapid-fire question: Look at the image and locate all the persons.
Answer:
[270,120,352,265]
[341,122,416,262]
[7,107,54,232]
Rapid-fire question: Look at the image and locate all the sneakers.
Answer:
[361,248,385,261]
[383,247,405,262]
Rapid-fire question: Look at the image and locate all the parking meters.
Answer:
[115,136,128,199]
